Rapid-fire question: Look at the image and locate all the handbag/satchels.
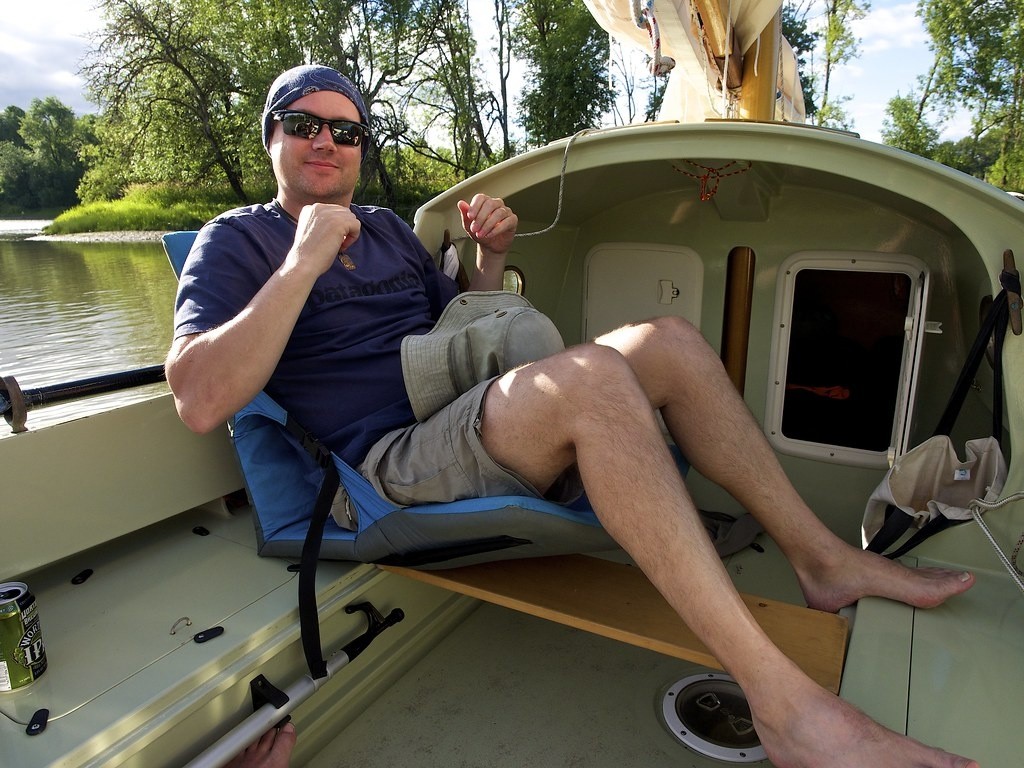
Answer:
[860,270,1020,557]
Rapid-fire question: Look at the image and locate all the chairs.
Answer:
[162,230,691,564]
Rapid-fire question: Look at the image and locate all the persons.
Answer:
[165,65,980,768]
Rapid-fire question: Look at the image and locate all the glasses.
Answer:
[272,111,371,147]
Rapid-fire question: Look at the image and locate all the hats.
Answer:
[402,291,563,423]
[262,64,370,167]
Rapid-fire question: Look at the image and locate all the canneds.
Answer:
[0,582,47,693]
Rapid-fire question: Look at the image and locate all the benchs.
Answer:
[0,391,472,768]
[838,553,1024,768]
[380,552,849,695]
